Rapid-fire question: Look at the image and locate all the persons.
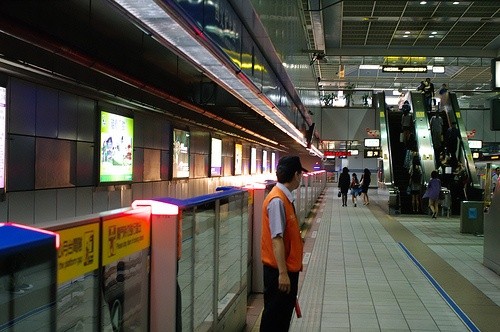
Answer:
[408,160,424,185]
[409,169,422,213]
[402,109,412,134]
[439,84,449,109]
[351,173,360,207]
[404,133,418,168]
[338,167,350,207]
[360,168,371,205]
[306,110,313,135]
[430,111,444,149]
[444,121,461,157]
[422,171,442,220]
[439,147,473,216]
[492,167,500,194]
[259,156,309,332]
[424,78,435,113]
[401,100,411,113]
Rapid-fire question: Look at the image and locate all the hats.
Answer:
[278,156,309,173]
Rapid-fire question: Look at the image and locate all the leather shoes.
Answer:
[431,211,437,220]
[342,204,347,207]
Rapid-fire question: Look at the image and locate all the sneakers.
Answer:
[362,201,369,205]
[353,201,356,206]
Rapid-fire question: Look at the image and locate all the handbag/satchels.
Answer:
[412,165,421,184]
[359,175,364,185]
[338,189,342,197]
[399,131,405,142]
